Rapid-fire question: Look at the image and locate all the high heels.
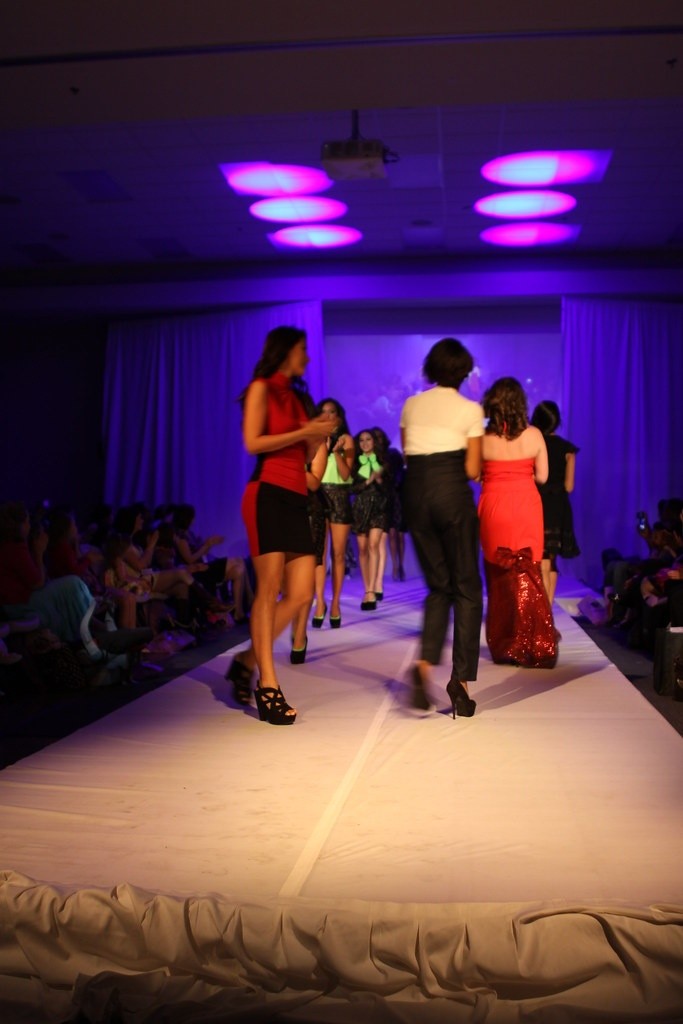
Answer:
[330,607,341,629]
[359,591,377,610]
[254,678,298,725]
[405,665,438,711]
[374,586,384,601]
[224,656,254,708]
[311,602,327,628]
[445,677,477,721]
[289,633,308,664]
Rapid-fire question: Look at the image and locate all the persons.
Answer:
[221,326,342,727]
[349,425,405,611]
[475,375,562,671]
[1,460,258,724]
[308,397,354,631]
[580,499,683,671]
[522,400,581,614]
[393,338,488,722]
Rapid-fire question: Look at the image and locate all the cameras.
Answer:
[637,511,648,529]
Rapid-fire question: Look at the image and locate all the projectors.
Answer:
[320,139,388,180]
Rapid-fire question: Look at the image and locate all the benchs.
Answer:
[104,567,181,633]
